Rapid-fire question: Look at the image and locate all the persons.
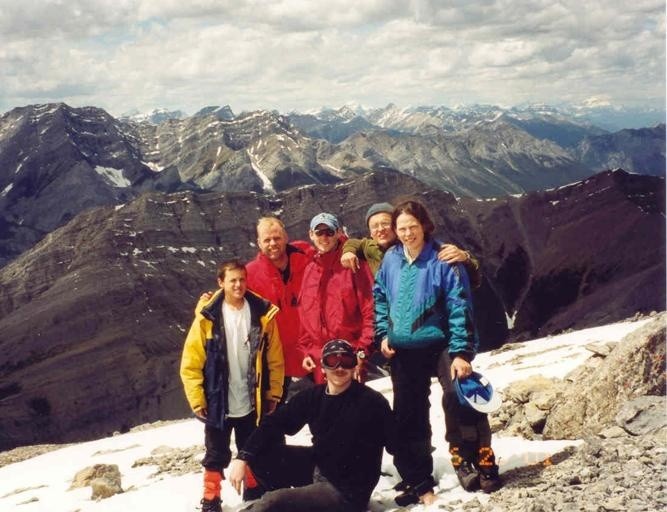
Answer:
[341,202,480,491]
[200,216,317,405]
[372,200,499,499]
[180,260,285,512]
[298,213,376,383]
[229,339,437,511]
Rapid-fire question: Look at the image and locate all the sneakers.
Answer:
[454,463,500,492]
[395,475,434,506]
[202,496,222,512]
[244,485,264,501]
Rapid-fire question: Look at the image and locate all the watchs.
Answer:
[357,352,368,362]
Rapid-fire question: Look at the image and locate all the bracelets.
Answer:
[464,250,471,264]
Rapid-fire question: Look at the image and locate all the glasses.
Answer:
[315,228,335,236]
[322,351,355,369]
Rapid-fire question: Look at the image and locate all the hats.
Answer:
[310,213,339,231]
[453,371,501,413]
[322,339,354,354]
[366,202,396,228]
